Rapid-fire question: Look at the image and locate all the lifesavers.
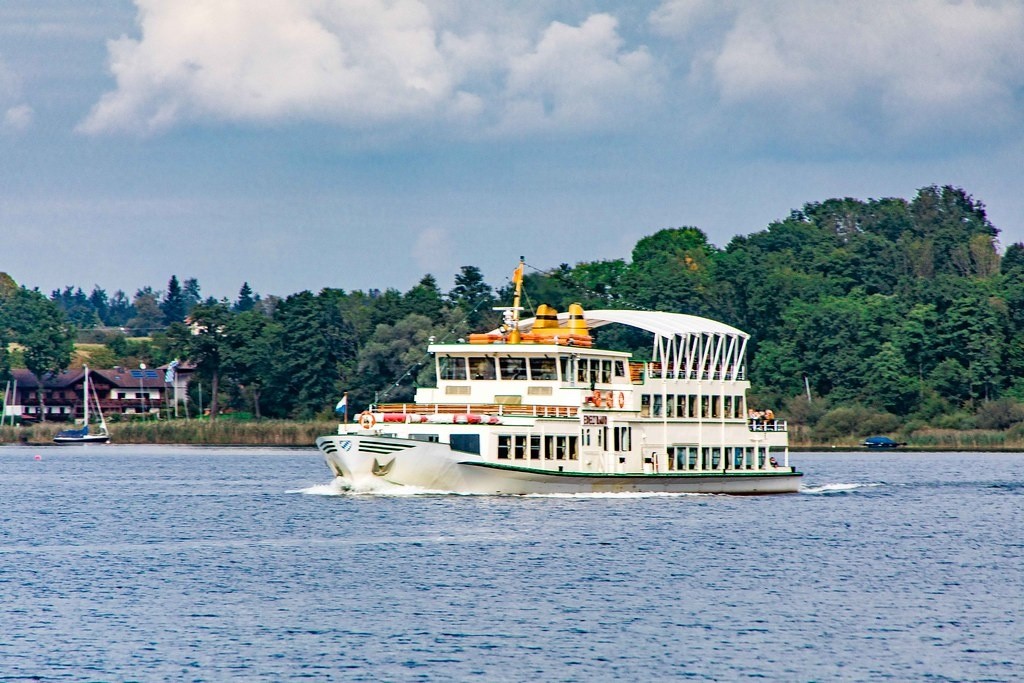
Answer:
[618,392,625,408]
[359,411,375,429]
[606,390,613,408]
[593,390,601,407]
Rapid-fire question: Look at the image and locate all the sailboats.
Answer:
[52,364,111,443]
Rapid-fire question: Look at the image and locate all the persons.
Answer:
[705,353,710,370]
[677,449,683,469]
[748,409,774,429]
[770,457,778,467]
[478,359,494,378]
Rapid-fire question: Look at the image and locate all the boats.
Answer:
[861,437,907,448]
[316,255,806,495]
